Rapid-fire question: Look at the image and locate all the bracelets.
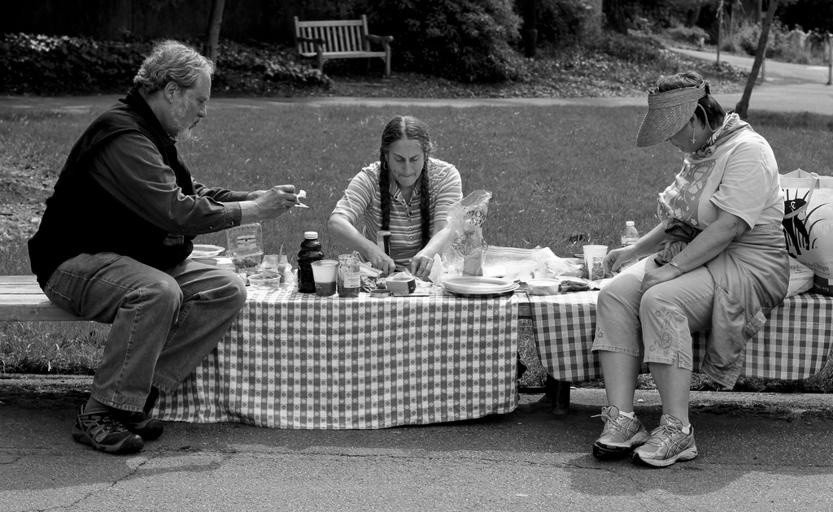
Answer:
[668,261,688,274]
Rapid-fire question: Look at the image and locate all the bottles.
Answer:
[620,220,639,247]
[296,231,323,293]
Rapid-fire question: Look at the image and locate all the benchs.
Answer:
[0,274,832,430]
[290,11,395,81]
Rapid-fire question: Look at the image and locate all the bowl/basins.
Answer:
[525,278,559,294]
[248,273,280,292]
[279,264,292,284]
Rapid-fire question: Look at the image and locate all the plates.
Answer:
[444,276,519,296]
[187,243,225,259]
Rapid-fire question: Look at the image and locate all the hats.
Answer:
[633,78,707,149]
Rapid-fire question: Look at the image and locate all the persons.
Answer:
[591,72,793,469]
[26,40,300,455]
[327,114,461,279]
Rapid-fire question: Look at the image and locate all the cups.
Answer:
[309,259,338,297]
[581,244,608,281]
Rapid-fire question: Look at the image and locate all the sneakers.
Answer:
[71,400,144,456]
[630,413,699,470]
[590,406,650,462]
[110,408,164,442]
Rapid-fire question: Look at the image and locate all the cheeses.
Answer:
[387,276,415,295]
